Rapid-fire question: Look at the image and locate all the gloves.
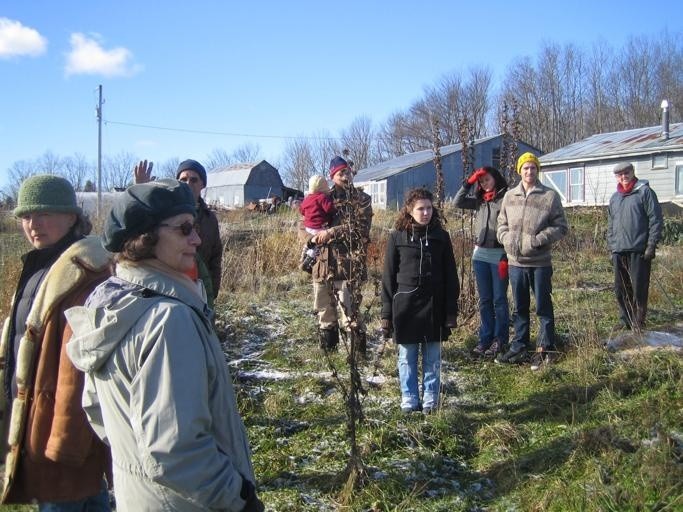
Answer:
[498,256,508,280]
[608,252,613,266]
[644,245,655,261]
[241,481,264,512]
[467,167,486,185]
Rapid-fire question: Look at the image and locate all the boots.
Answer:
[298,256,317,274]
[299,253,307,263]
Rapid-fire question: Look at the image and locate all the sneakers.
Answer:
[484,341,510,355]
[493,348,528,364]
[472,339,494,353]
[530,352,547,371]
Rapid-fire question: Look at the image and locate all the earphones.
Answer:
[410,237,414,242]
[424,240,429,246]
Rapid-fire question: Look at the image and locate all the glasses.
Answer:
[156,221,200,236]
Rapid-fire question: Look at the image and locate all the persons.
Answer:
[312,156,375,361]
[496,152,568,371]
[606,162,665,330]
[0,173,113,510]
[381,189,460,414]
[453,166,509,354]
[132,159,222,299]
[64,179,265,511]
[298,175,332,274]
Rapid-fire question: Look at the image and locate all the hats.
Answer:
[308,175,329,194]
[100,178,195,252]
[329,156,347,180]
[13,175,82,218]
[176,159,206,187]
[613,162,633,174]
[516,152,540,176]
